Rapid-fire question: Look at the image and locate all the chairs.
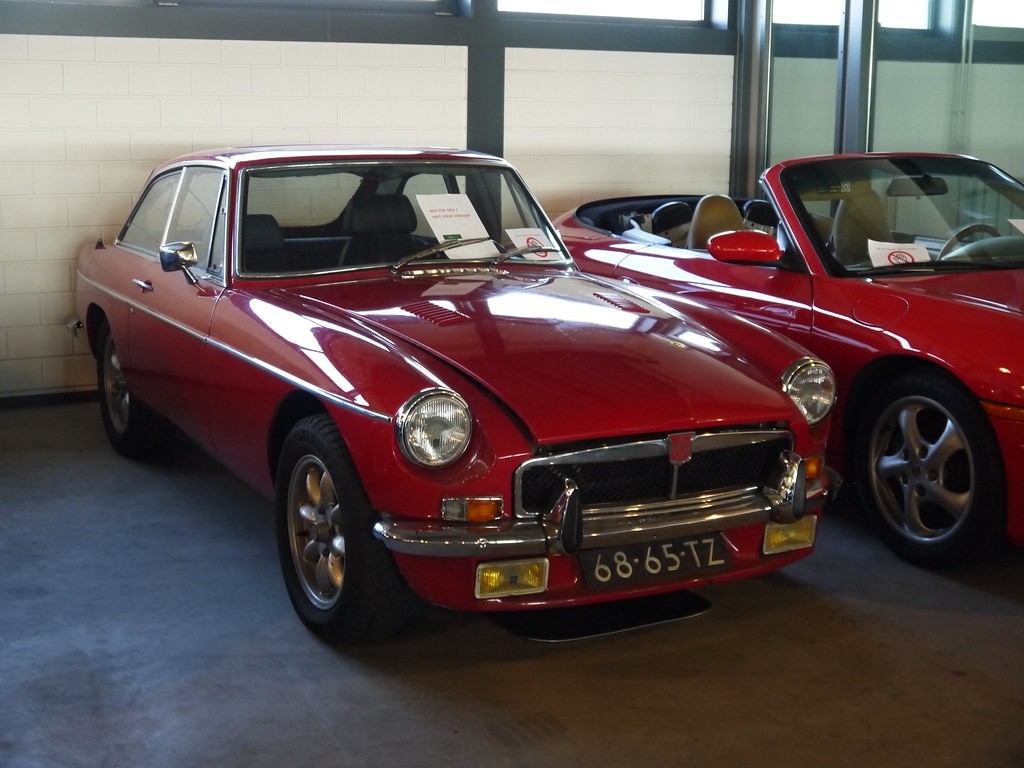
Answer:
[687,192,743,248]
[831,189,894,266]
[338,194,426,268]
[742,199,779,235]
[651,200,693,241]
[243,213,304,272]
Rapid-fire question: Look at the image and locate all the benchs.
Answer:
[283,237,349,269]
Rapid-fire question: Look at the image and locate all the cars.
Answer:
[72,147,838,646]
[546,149,1024,566]
